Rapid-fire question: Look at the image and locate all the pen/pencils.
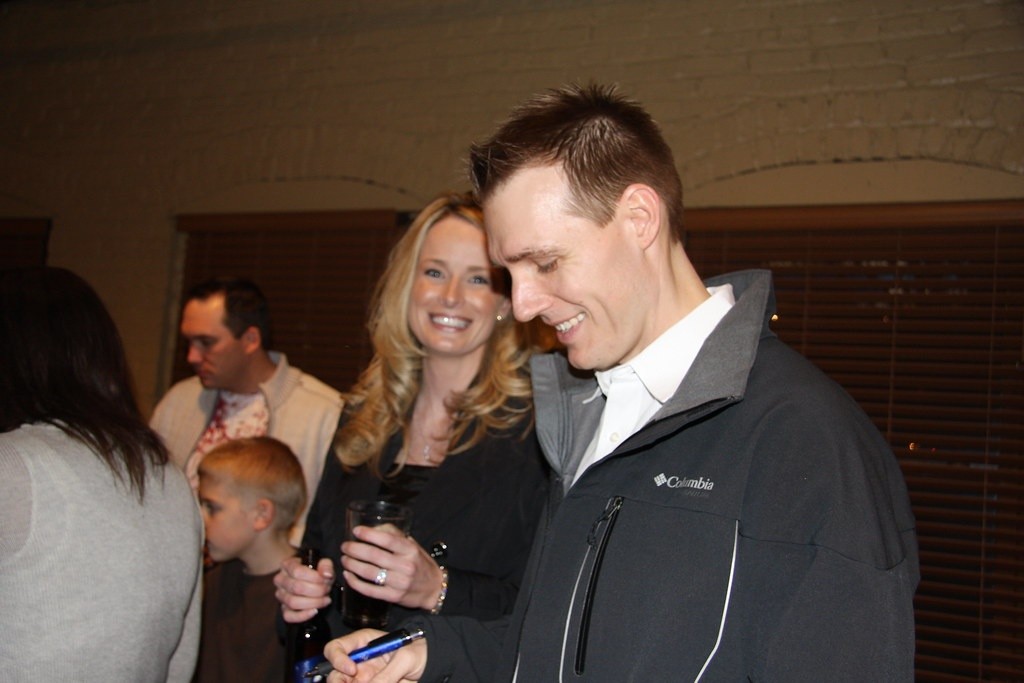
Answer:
[304,626,427,678]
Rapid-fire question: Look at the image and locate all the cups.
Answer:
[340,499,415,629]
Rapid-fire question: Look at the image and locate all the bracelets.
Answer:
[430,565,448,615]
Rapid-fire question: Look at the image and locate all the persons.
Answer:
[0,267,206,683]
[273,191,552,683]
[189,437,308,683]
[148,280,344,549]
[323,81,920,683]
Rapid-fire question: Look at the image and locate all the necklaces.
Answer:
[416,418,436,455]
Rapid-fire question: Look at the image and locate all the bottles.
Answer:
[282,548,331,683]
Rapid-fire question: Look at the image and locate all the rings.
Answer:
[374,568,388,585]
